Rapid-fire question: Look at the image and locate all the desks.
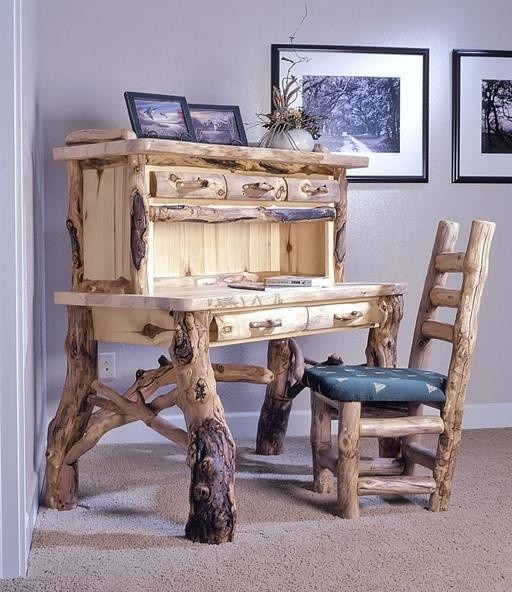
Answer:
[39,283,406,545]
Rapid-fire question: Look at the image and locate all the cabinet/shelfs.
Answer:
[51,137,369,296]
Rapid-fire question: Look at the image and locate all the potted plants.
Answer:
[250,3,331,150]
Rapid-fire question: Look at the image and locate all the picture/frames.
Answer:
[123,91,196,142]
[271,44,430,183]
[188,102,248,146]
[452,50,512,183]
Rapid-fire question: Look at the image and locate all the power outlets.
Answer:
[97,352,117,380]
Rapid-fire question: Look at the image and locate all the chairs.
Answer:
[301,218,498,519]
